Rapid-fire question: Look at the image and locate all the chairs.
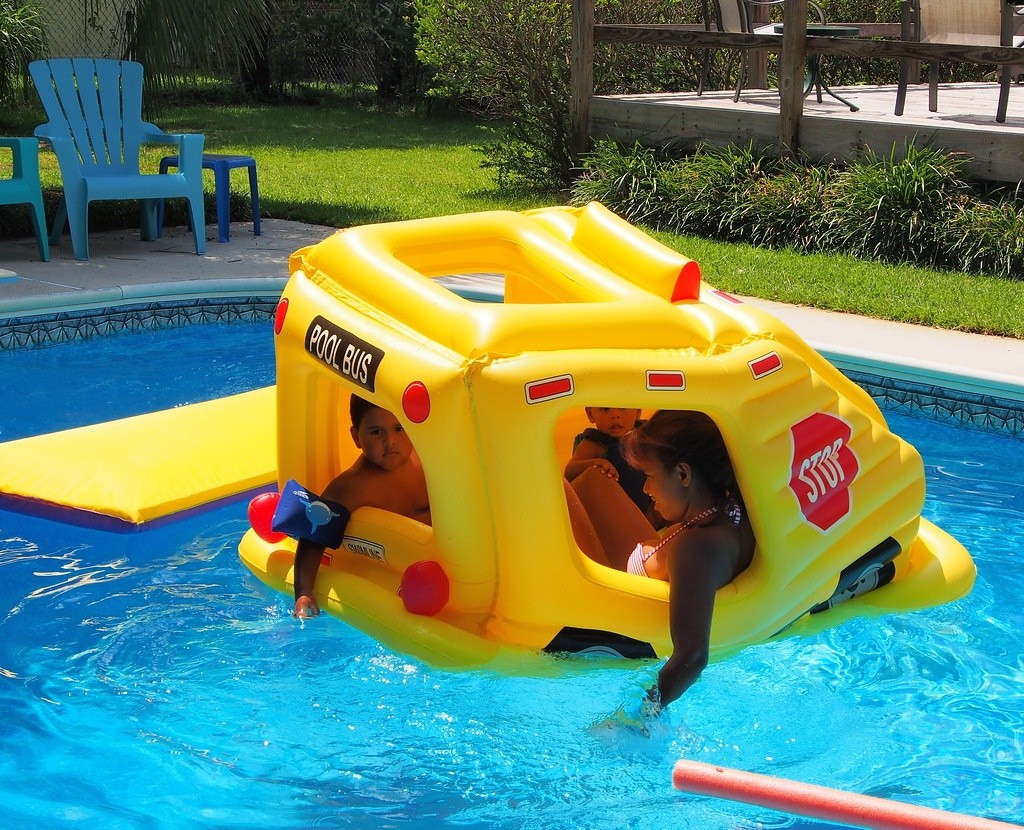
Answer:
[894,0,1024,123]
[0,137,49,262]
[29,58,207,260]
[697,0,825,104]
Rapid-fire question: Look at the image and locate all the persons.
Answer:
[562,407,755,702]
[565,407,677,530]
[293,393,432,611]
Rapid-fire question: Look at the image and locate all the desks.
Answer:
[774,24,860,112]
[158,154,261,242]
